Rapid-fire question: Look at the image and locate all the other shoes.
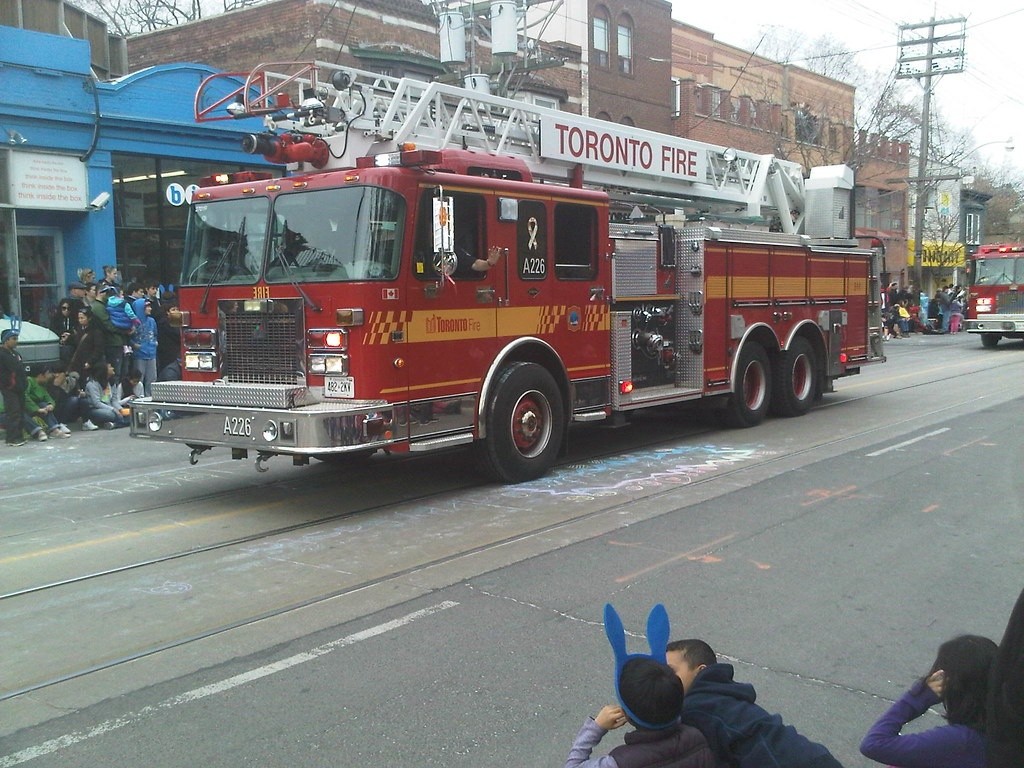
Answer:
[104,421,114,429]
[37,430,46,441]
[124,346,133,357]
[49,429,70,438]
[6,436,28,446]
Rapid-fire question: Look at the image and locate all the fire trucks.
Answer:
[959,243,1024,347]
[122,57,889,493]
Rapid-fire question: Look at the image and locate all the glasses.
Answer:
[62,306,72,312]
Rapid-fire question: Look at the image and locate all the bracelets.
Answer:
[487,260,495,267]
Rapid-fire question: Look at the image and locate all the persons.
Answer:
[666,638,842,768]
[0,316,28,446]
[858,635,997,768]
[928,284,969,334]
[883,282,925,341]
[0,363,71,441]
[48,363,98,433]
[53,266,183,429]
[565,656,717,768]
[986,589,1024,768]
[414,217,502,278]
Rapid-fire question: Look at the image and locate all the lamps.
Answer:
[8,129,27,146]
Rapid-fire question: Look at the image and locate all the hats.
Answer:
[69,282,87,290]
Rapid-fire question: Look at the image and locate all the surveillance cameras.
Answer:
[90,191,110,208]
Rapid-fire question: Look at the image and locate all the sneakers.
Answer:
[59,423,71,433]
[82,420,98,431]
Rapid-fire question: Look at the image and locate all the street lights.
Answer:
[910,137,1016,307]
